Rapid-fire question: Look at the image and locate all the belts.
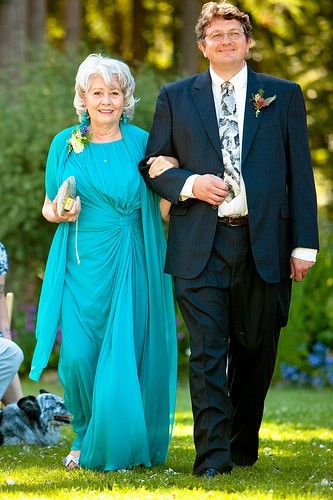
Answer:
[217,216,247,226]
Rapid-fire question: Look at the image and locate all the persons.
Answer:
[42,54,178,472]
[0,242,22,408]
[137,1,319,475]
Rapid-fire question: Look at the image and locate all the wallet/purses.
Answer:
[56,176,75,217]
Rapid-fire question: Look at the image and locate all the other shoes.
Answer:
[201,468,218,477]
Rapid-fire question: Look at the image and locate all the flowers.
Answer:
[249,87,276,117]
[65,126,93,161]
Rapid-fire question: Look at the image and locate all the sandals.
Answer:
[64,451,79,471]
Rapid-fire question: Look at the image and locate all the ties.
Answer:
[218,81,242,205]
